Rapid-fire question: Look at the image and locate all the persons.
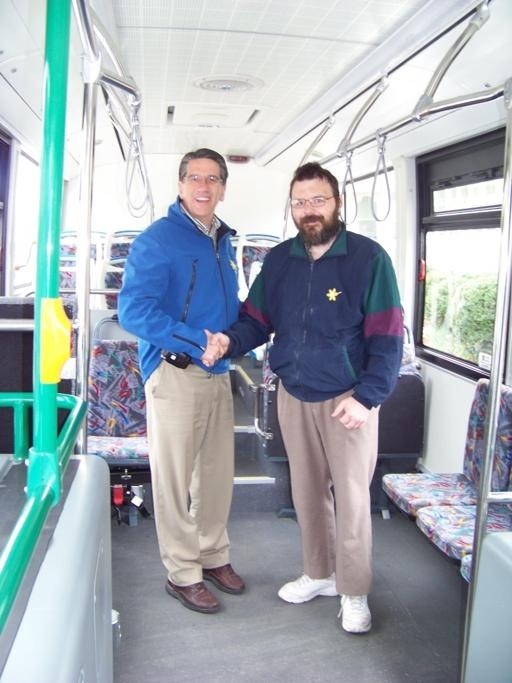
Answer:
[198,161,406,635]
[117,148,247,613]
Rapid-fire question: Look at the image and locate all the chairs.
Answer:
[459,554,474,582]
[19,229,425,526]
[416,484,512,558]
[382,376,511,514]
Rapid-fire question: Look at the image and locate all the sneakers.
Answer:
[278,572,340,605]
[339,592,372,633]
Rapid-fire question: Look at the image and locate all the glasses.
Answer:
[182,173,226,187]
[291,195,335,208]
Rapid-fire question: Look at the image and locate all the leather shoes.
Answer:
[166,578,220,613]
[201,563,246,594]
